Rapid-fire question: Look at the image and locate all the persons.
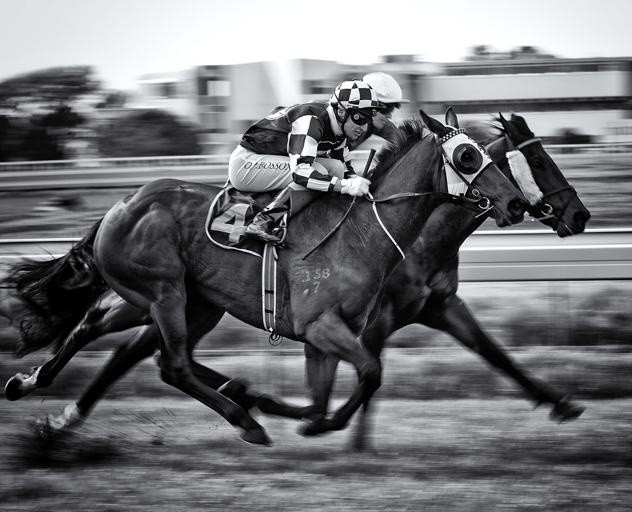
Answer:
[226,79,385,249]
[346,72,412,153]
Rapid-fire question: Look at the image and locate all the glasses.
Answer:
[350,110,372,125]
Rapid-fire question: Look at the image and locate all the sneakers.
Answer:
[245,221,280,241]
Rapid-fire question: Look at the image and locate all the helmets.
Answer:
[364,73,410,104]
[332,80,387,110]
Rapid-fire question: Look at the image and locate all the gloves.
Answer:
[341,176,370,196]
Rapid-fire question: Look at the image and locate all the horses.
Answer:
[1,105,592,456]
